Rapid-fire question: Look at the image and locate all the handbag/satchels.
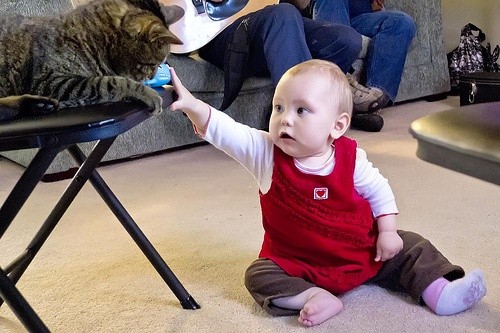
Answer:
[447,23,500,96]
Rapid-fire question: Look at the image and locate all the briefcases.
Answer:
[460,72,500,106]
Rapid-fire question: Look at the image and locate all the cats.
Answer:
[0,0,185,124]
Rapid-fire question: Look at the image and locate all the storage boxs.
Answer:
[460,72,500,106]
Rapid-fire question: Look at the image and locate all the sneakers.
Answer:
[345,72,384,132]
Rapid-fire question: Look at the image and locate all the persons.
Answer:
[169,58,486,328]
[196,0,418,133]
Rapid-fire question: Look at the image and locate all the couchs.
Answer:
[0,0,451,182]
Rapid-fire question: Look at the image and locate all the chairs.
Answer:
[0,86,201,333]
